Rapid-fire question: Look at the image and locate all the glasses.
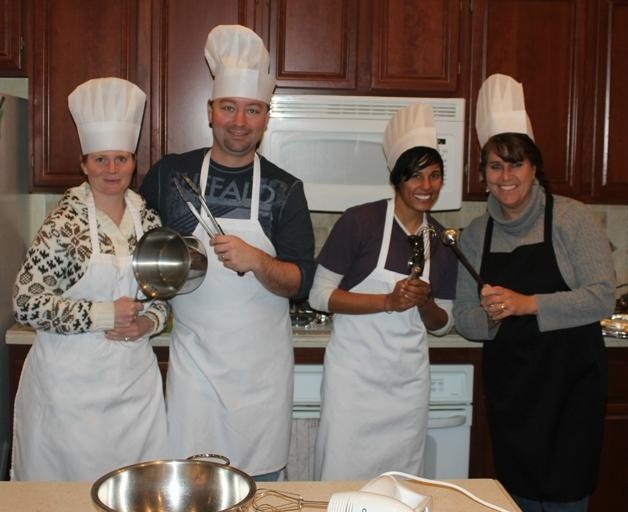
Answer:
[408,235,425,276]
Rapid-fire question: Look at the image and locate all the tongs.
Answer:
[172,175,246,277]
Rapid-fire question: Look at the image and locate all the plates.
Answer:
[599,313,628,339]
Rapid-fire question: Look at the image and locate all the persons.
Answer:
[135,97,316,482]
[10,148,171,484]
[448,132,616,512]
[306,146,459,481]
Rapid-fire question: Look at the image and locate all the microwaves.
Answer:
[257,93,468,215]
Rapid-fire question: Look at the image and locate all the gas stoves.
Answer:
[284,297,333,332]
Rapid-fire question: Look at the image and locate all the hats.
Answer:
[475,73,535,150]
[382,104,438,172]
[204,24,276,105]
[68,77,147,156]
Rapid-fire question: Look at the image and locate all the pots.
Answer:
[131,226,208,303]
[88,452,258,511]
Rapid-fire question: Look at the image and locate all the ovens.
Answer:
[294,363,476,481]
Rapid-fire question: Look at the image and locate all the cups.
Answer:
[326,489,413,512]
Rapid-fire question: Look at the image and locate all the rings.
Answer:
[123,335,130,343]
[500,303,505,311]
[220,253,225,262]
